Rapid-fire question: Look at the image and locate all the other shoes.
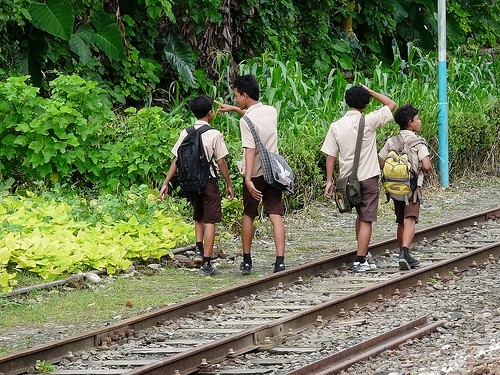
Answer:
[408,255,421,266]
[399,249,411,270]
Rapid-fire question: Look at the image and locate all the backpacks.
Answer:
[177,124,211,195]
[383,135,427,205]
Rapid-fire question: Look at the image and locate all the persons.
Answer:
[378,105,431,268]
[321,83,396,271]
[160,95,236,276]
[218,74,286,275]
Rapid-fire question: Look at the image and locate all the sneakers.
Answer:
[198,267,215,275]
[273,264,285,273]
[354,261,373,272]
[366,252,377,269]
[240,262,252,274]
[194,253,203,269]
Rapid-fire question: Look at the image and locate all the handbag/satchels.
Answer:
[261,153,293,191]
[335,176,361,213]
